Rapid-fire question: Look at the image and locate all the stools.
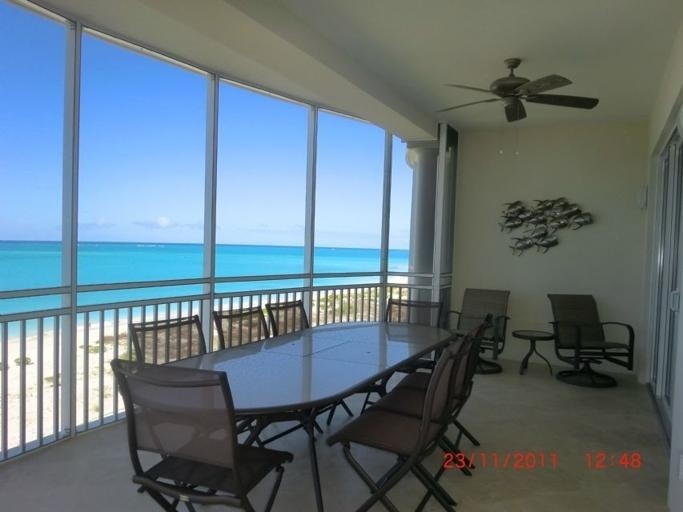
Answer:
[514,331,555,377]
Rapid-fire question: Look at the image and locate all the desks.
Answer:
[162,321,451,512]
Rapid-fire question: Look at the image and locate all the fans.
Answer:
[435,58,599,123]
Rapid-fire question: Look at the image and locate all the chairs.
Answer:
[110,360,293,512]
[384,298,442,377]
[266,302,353,425]
[327,350,458,512]
[211,308,271,349]
[361,334,475,474]
[547,293,634,389]
[129,316,205,364]
[444,289,510,374]
[452,312,492,447]
